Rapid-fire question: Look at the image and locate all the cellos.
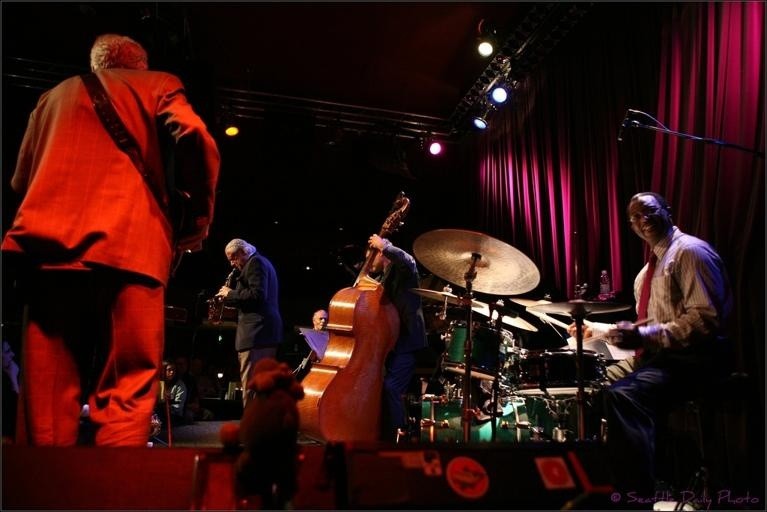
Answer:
[295,190,411,447]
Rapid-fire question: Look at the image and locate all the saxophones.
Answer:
[205,266,237,329]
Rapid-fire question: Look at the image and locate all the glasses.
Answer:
[629,206,663,224]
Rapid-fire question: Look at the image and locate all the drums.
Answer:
[440,319,516,380]
[406,393,593,444]
[512,348,608,395]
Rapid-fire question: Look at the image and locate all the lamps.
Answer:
[472,16,521,129]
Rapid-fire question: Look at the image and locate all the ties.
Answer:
[635,253,656,364]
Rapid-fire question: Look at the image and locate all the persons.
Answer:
[295,306,329,375]
[3,32,223,450]
[219,239,280,416]
[362,236,424,444]
[567,192,731,495]
[1,329,203,445]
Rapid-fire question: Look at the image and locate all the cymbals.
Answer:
[408,229,632,332]
